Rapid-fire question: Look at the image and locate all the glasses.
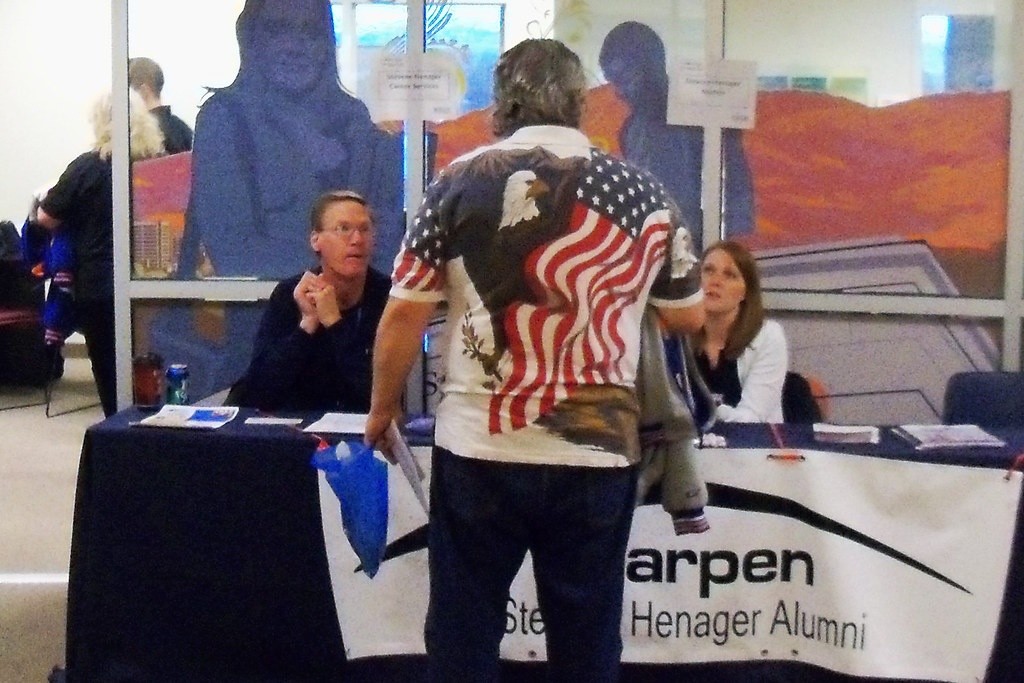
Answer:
[314,223,375,237]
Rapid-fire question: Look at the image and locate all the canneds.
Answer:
[165,364,188,406]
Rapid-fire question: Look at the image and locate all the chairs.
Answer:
[941,372,1024,426]
[0,221,50,412]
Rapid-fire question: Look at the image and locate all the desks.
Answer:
[64,401,1024,683]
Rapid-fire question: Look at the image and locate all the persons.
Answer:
[225,192,403,414]
[151,1,406,405]
[130,57,193,154]
[688,241,789,424]
[37,88,169,419]
[598,20,755,256]
[363,38,705,683]
